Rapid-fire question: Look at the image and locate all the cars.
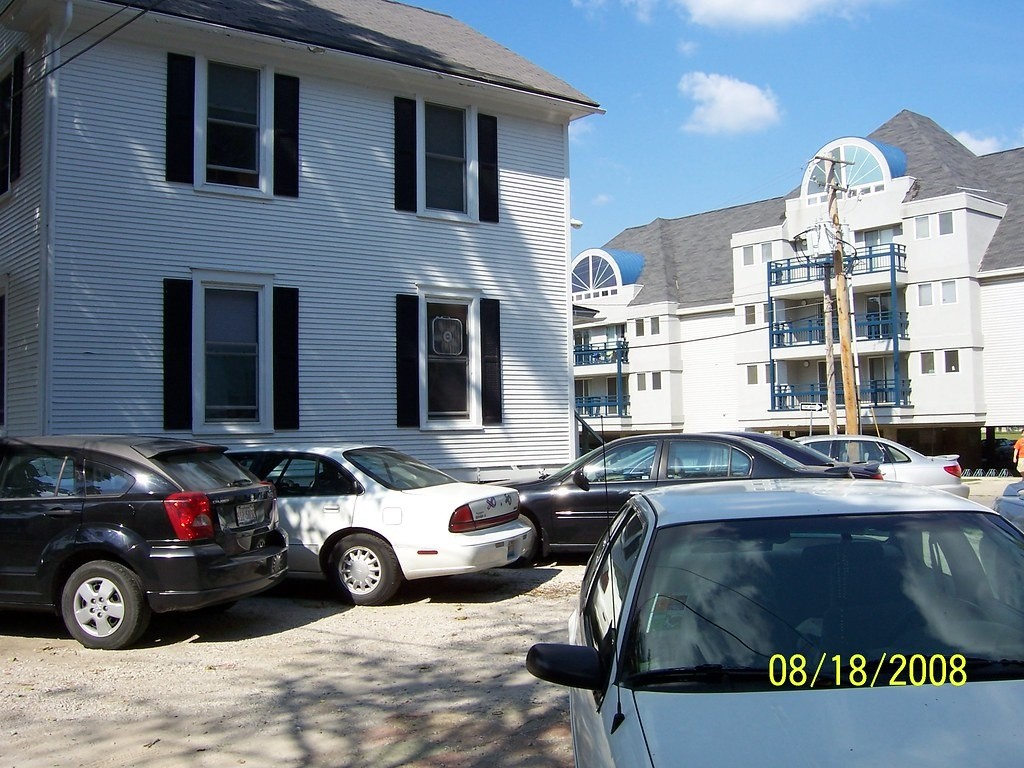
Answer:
[787,434,972,500]
[990,479,1024,534]
[221,441,536,607]
[0,430,292,652]
[696,430,885,484]
[480,432,858,568]
[523,473,1024,768]
[980,437,1014,458]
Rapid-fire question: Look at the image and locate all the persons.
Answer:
[1013,430,1024,482]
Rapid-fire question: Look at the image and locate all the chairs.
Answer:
[673,458,686,478]
[664,539,961,663]
